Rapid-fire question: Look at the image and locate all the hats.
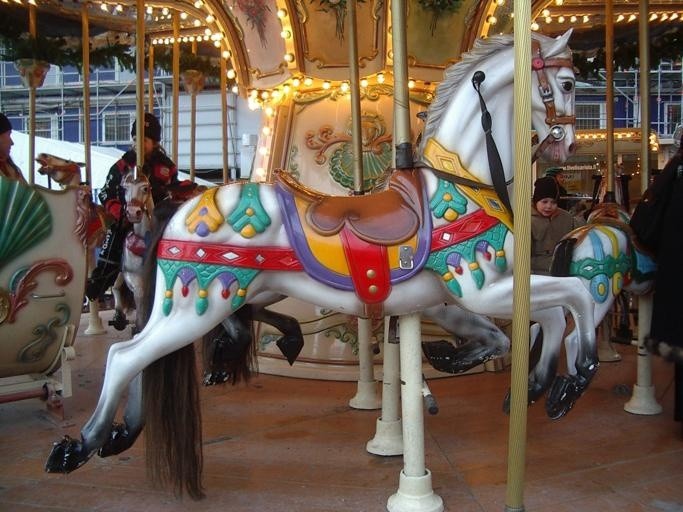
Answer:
[0,112,12,136]
[131,112,163,141]
[546,166,563,173]
[532,178,560,205]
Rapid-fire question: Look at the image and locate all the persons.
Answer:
[0,113,27,184]
[530,176,574,274]
[545,167,567,212]
[84,113,185,301]
[628,138,683,371]
